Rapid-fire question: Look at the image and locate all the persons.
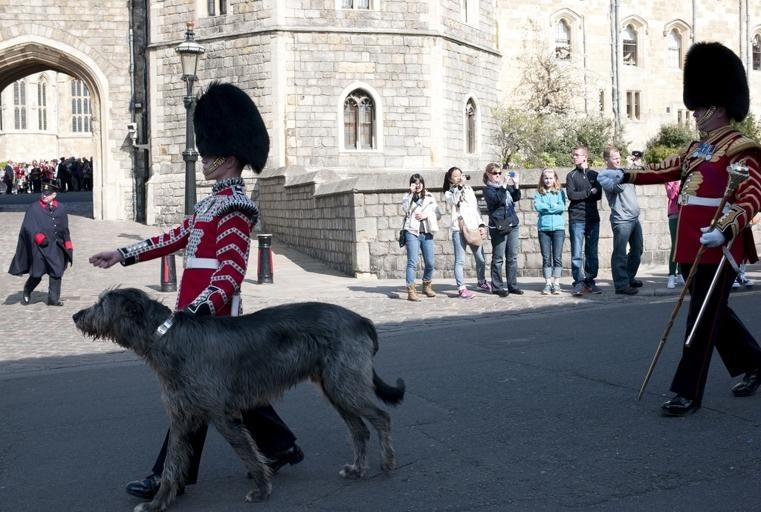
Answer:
[666,170,684,289]
[397,174,447,303]
[533,167,567,296]
[441,167,493,300]
[597,145,644,296]
[6,179,73,305]
[482,163,525,298]
[732,262,755,289]
[597,107,761,415]
[0,154,93,197]
[566,145,603,297]
[88,153,304,498]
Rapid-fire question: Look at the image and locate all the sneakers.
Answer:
[667,275,675,289]
[736,272,753,286]
[585,284,601,294]
[732,276,740,288]
[459,289,475,299]
[476,280,491,292]
[552,284,560,294]
[542,285,551,295]
[676,273,684,283]
[572,284,585,295]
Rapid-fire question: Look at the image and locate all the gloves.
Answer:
[40,238,48,247]
[699,226,725,248]
[596,169,624,191]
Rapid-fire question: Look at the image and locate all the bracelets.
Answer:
[458,181,464,190]
[479,224,486,228]
[411,191,415,194]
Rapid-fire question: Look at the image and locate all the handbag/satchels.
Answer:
[457,215,482,247]
[496,218,511,236]
[399,230,405,248]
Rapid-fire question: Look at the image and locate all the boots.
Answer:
[422,282,435,297]
[407,284,421,301]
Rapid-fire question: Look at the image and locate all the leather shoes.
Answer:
[47,302,63,305]
[492,291,508,296]
[512,289,523,294]
[615,287,638,295]
[660,394,701,416]
[124,473,184,500]
[22,291,29,303]
[629,279,642,287]
[246,444,303,479]
[730,374,761,397]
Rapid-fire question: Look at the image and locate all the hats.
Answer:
[193,80,269,174]
[40,182,58,195]
[683,41,749,123]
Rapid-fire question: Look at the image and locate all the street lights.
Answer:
[174,16,207,218]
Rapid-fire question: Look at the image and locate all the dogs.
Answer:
[73,284,406,512]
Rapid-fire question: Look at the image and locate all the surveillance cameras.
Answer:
[126,123,138,141]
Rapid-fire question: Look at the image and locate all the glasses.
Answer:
[488,171,501,175]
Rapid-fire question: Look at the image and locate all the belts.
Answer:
[677,194,722,208]
[182,254,218,269]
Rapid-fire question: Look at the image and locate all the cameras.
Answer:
[632,151,643,159]
[464,175,470,180]
[412,183,419,188]
[508,172,516,178]
